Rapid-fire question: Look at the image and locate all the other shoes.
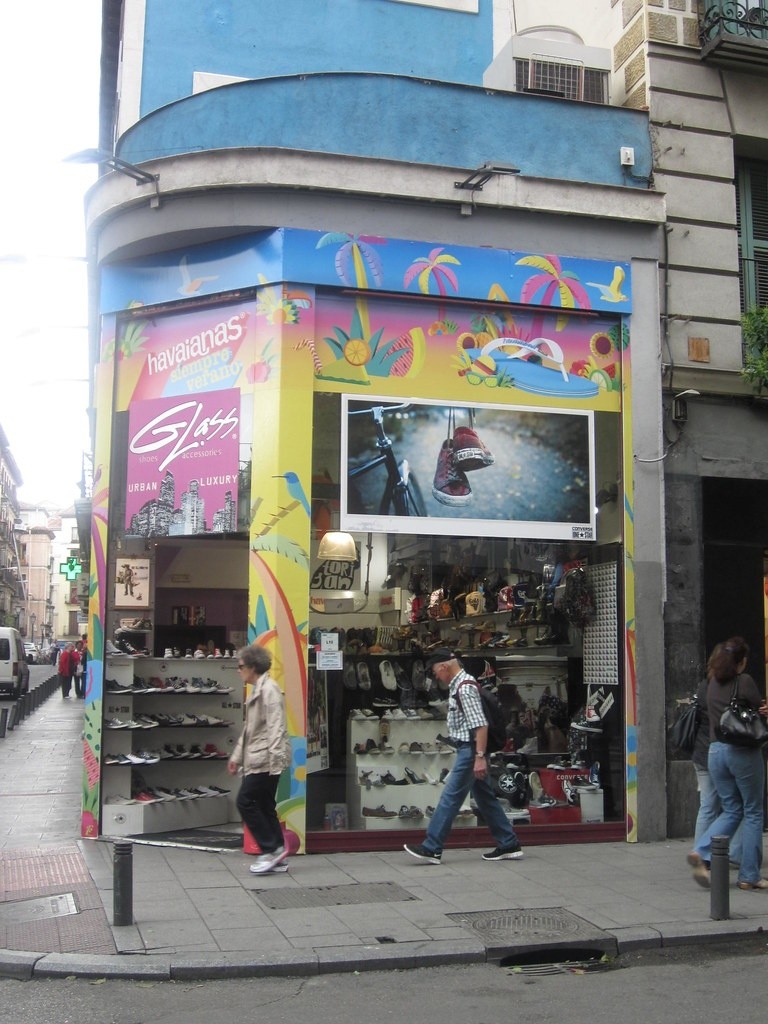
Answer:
[730,864,740,870]
[686,850,711,888]
[78,694,85,699]
[737,876,768,890]
[701,860,711,871]
[64,695,72,699]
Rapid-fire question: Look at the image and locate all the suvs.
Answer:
[23,642,39,665]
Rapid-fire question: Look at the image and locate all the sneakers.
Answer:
[453,407,495,471]
[432,406,474,507]
[268,860,289,872]
[482,841,523,860]
[250,847,287,872]
[102,601,603,825]
[404,843,442,864]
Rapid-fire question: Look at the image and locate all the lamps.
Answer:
[68,149,159,185]
[454,162,521,191]
[317,532,356,561]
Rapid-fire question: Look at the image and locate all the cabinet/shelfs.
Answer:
[308,604,575,833]
[103,653,246,834]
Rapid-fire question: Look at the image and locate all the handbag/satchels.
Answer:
[76,660,83,677]
[718,673,768,749]
[666,700,700,753]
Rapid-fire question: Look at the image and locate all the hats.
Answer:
[423,648,456,672]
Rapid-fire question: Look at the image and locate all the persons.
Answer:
[228,645,291,873]
[685,636,768,890]
[403,647,524,863]
[195,642,208,657]
[50,643,56,666]
[59,640,87,698]
[124,565,133,595]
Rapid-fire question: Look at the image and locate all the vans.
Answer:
[0,627,33,700]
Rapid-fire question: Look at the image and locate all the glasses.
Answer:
[238,663,246,670]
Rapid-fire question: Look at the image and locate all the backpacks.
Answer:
[456,680,507,754]
[404,558,596,623]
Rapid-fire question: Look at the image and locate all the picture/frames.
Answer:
[110,553,155,609]
